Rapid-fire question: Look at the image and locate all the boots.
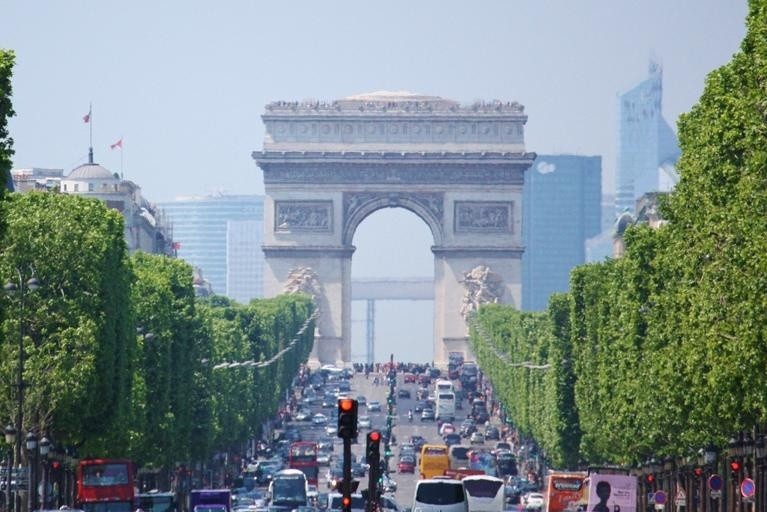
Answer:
[327,468,343,490]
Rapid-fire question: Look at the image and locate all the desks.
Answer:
[3,262,41,465]
[25,431,37,511]
[5,423,16,511]
[39,436,48,511]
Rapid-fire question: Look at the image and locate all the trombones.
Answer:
[648,493,654,503]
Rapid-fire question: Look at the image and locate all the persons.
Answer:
[591,481,612,512]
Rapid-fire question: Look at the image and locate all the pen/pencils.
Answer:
[327,494,406,512]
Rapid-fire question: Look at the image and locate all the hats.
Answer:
[396,362,544,512]
[358,415,371,428]
[373,428,395,444]
[356,396,366,405]
[382,474,398,493]
[230,408,338,511]
[301,366,354,408]
[367,400,380,411]
[329,452,372,477]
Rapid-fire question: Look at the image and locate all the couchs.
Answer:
[646,474,652,482]
[730,462,738,485]
[348,481,359,494]
[338,398,356,438]
[366,432,379,463]
[52,460,59,472]
[341,494,351,510]
[694,467,702,481]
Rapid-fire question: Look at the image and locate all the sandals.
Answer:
[75,458,135,511]
[544,474,585,511]
[137,468,174,492]
[587,464,630,477]
